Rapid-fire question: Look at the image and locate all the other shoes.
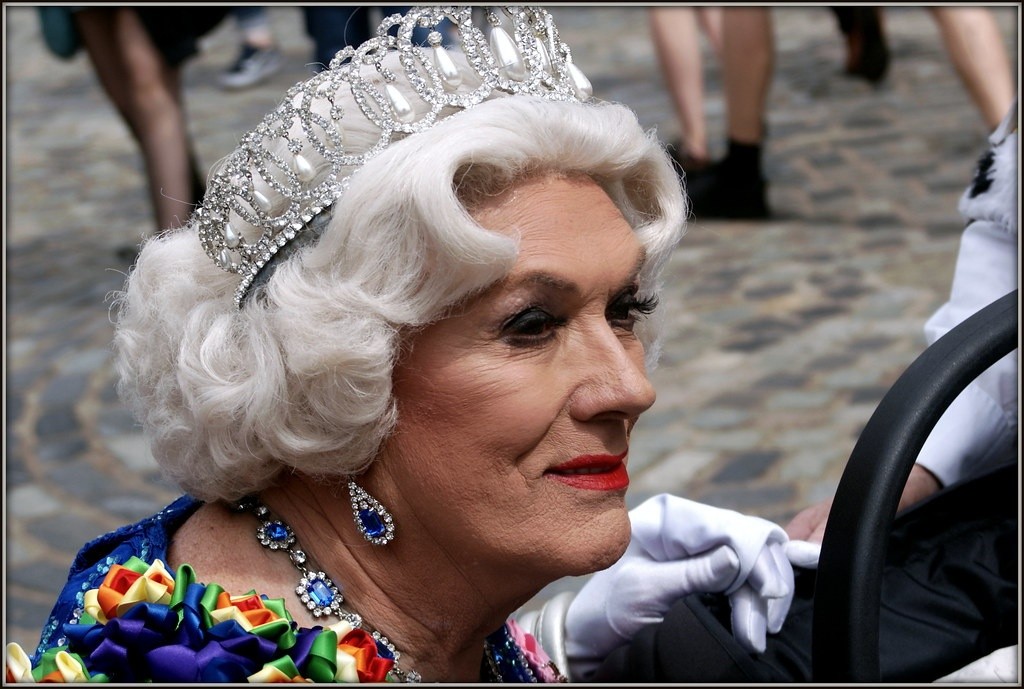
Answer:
[671,146,771,219]
[218,42,285,87]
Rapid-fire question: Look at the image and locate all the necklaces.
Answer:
[220,480,546,684]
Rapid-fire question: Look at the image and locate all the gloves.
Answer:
[560,492,822,661]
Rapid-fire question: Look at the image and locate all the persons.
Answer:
[782,100,1018,546]
[218,6,489,92]
[648,6,1018,225]
[38,6,235,264]
[6,6,822,683]
